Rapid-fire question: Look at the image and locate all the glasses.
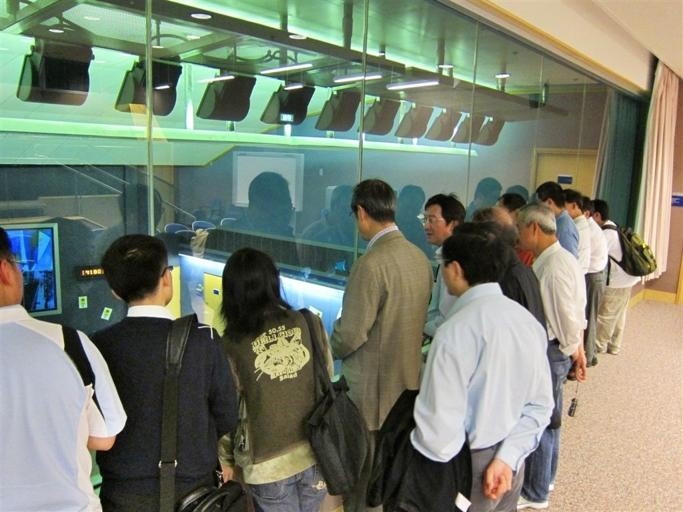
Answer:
[418,218,444,225]
[16,260,25,272]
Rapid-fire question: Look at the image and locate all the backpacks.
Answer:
[601,220,657,286]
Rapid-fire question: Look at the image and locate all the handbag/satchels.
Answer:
[305,389,369,495]
[177,481,254,512]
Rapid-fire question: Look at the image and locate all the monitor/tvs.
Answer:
[0,222,62,317]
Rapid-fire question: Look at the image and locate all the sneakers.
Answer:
[517,495,548,510]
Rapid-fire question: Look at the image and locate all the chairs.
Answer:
[164,215,237,243]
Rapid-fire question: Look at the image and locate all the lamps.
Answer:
[196,71,256,122]
[358,97,401,135]
[14,36,94,107]
[316,85,362,131]
[424,108,461,141]
[453,112,485,143]
[473,117,505,145]
[114,52,183,117]
[261,80,315,126]
[394,104,434,139]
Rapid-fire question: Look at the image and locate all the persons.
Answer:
[536,181,634,367]
[409,221,554,512]
[398,185,435,265]
[216,247,335,511]
[472,206,562,432]
[89,230,238,511]
[507,184,528,203]
[494,192,536,267]
[0,225,130,511]
[89,182,182,270]
[420,192,466,395]
[328,177,434,511]
[304,186,372,275]
[515,202,589,509]
[467,176,502,225]
[206,170,299,268]
